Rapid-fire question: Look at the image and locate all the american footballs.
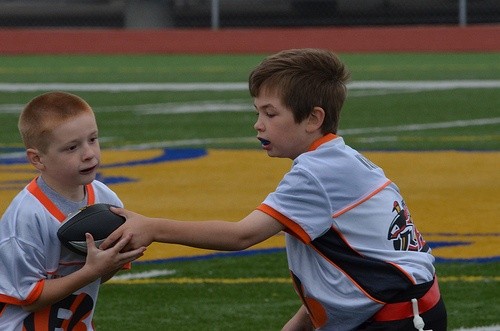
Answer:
[56,203,126,255]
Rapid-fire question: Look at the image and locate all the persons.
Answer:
[0,92,145,331]
[100,48,451,331]
